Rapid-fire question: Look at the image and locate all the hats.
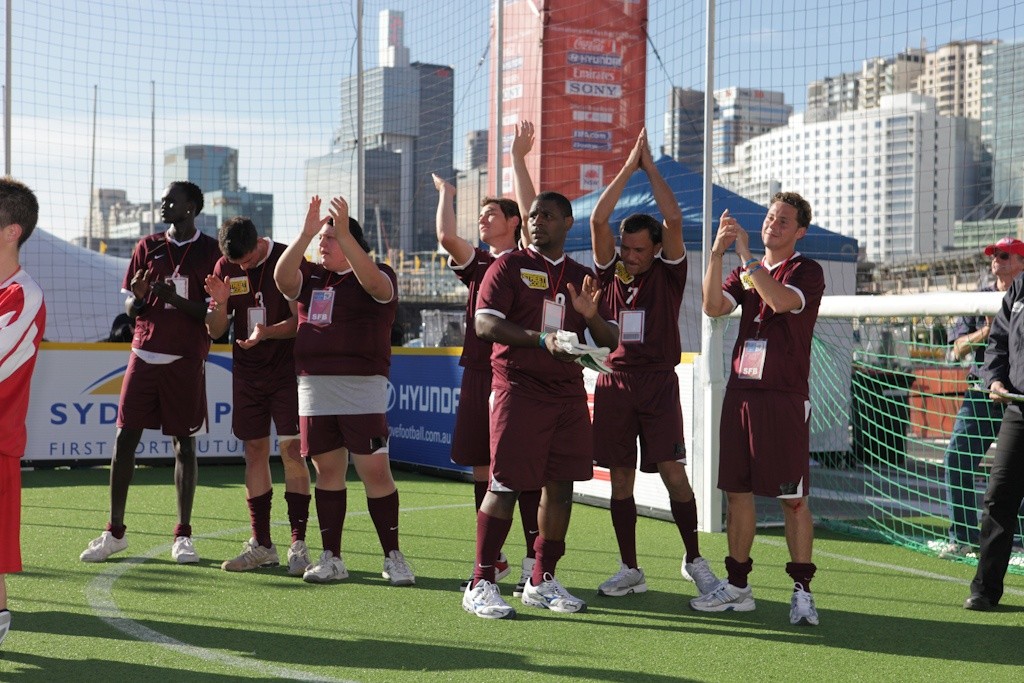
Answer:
[985,238,1024,256]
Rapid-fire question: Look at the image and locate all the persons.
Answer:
[204,217,313,578]
[433,121,543,597]
[77,180,223,562]
[690,191,825,626]
[590,127,722,598]
[461,191,622,620]
[274,195,417,585]
[945,237,1024,610]
[0,176,46,645]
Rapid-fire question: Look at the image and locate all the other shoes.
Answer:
[939,542,973,558]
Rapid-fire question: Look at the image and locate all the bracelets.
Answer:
[539,332,550,348]
[743,258,762,277]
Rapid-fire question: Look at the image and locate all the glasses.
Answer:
[993,252,1011,259]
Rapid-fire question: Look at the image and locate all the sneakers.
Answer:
[681,553,721,595]
[513,558,537,597]
[964,593,994,611]
[381,550,415,585]
[462,578,516,619]
[790,582,820,625]
[690,579,755,612]
[287,540,311,575]
[520,572,587,612]
[460,552,510,592]
[598,560,647,596]
[302,550,349,582]
[80,531,128,562]
[221,537,279,571]
[172,536,200,562]
